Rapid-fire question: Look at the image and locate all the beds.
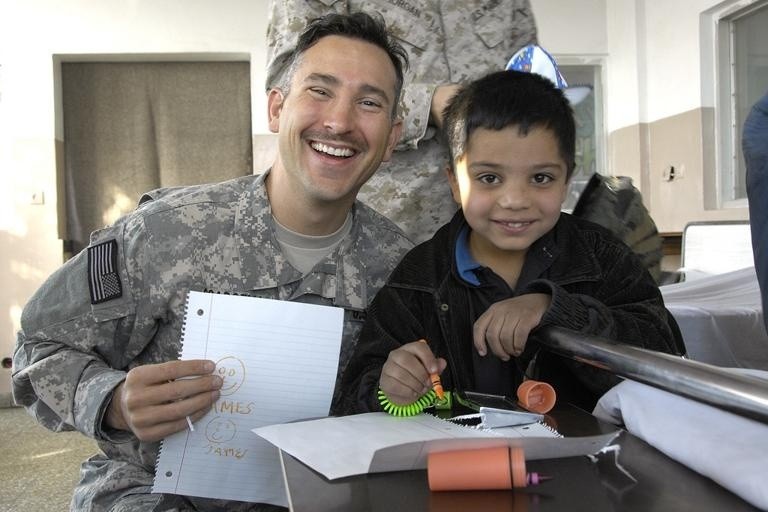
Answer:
[657,220,766,370]
[522,322,766,512]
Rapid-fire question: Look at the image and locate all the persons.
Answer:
[330,70,687,416]
[10,11,417,512]
[265,0,539,247]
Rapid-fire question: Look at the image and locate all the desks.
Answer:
[278,390,765,512]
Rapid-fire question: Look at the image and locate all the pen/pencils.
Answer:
[419,338,444,400]
[526,472,556,486]
